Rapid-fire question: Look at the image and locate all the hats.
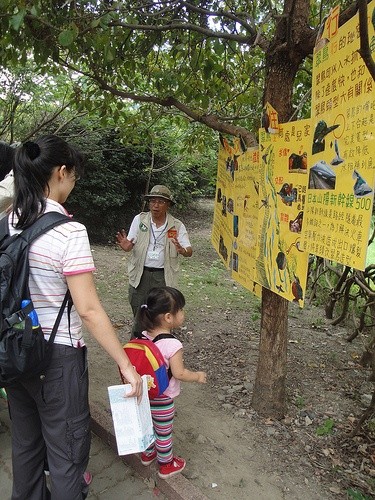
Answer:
[144,184,176,206]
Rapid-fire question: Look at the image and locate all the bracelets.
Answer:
[184,248,188,253]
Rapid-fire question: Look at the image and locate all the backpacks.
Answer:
[119,333,177,399]
[0,212,81,388]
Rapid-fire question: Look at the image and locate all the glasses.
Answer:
[149,199,169,204]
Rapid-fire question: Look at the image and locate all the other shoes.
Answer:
[157,457,186,478]
[82,470,92,484]
[141,450,157,465]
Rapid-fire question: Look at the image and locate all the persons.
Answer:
[0,134,143,500]
[131,287,207,478]
[115,185,192,319]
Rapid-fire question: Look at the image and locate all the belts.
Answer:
[144,266,164,272]
